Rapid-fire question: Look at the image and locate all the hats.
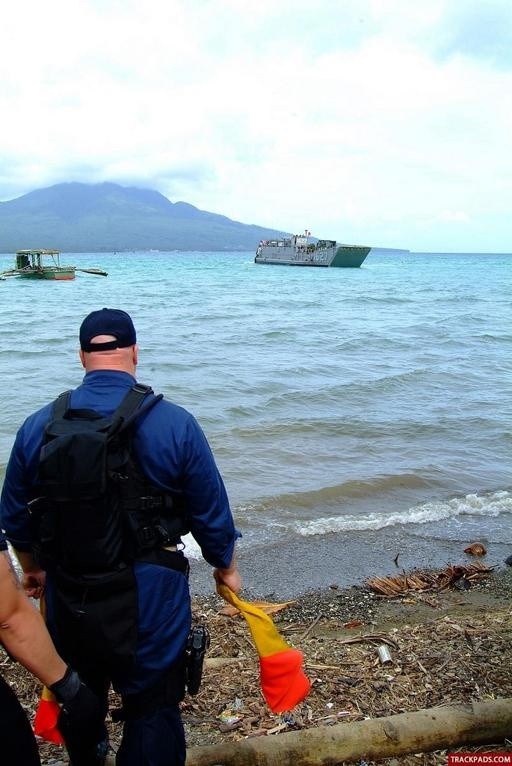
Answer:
[80,308,136,352]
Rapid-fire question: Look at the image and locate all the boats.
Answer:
[14,250,75,278]
[255,236,370,267]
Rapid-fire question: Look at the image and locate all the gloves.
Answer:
[49,662,108,747]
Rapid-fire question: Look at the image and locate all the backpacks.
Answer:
[27,383,154,573]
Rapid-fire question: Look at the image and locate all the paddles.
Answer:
[57,267,108,275]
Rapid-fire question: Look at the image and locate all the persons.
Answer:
[0,527,94,764]
[2,306,245,763]
